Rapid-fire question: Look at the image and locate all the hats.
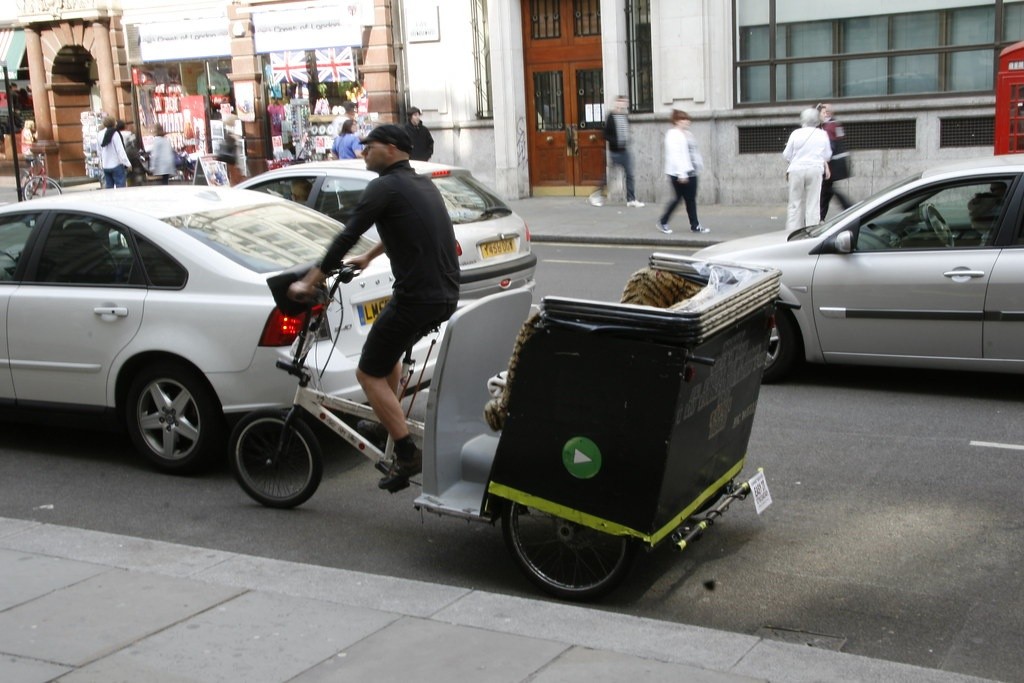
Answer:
[358,124,413,153]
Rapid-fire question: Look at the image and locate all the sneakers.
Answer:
[379,448,422,488]
[657,223,673,233]
[358,420,387,448]
[691,225,710,234]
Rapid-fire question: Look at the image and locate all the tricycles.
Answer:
[222,247,804,604]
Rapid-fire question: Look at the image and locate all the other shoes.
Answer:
[627,199,644,208]
[589,195,603,207]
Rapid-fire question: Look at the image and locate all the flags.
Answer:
[315,46,355,83]
[270,51,308,84]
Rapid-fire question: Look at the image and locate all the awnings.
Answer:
[0,28,29,80]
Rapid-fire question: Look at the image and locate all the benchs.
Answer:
[483,246,780,450]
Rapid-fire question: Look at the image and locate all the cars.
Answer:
[0,184,456,476]
[229,155,537,318]
[684,152,1024,385]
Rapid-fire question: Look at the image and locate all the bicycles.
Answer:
[20,152,63,202]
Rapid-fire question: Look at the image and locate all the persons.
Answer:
[149,123,176,184]
[330,101,363,159]
[402,107,434,162]
[967,192,1002,248]
[783,103,852,227]
[589,95,645,207]
[21,120,37,167]
[96,116,152,188]
[289,124,460,490]
[290,180,312,204]
[655,109,710,234]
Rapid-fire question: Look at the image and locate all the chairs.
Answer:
[63,219,118,283]
